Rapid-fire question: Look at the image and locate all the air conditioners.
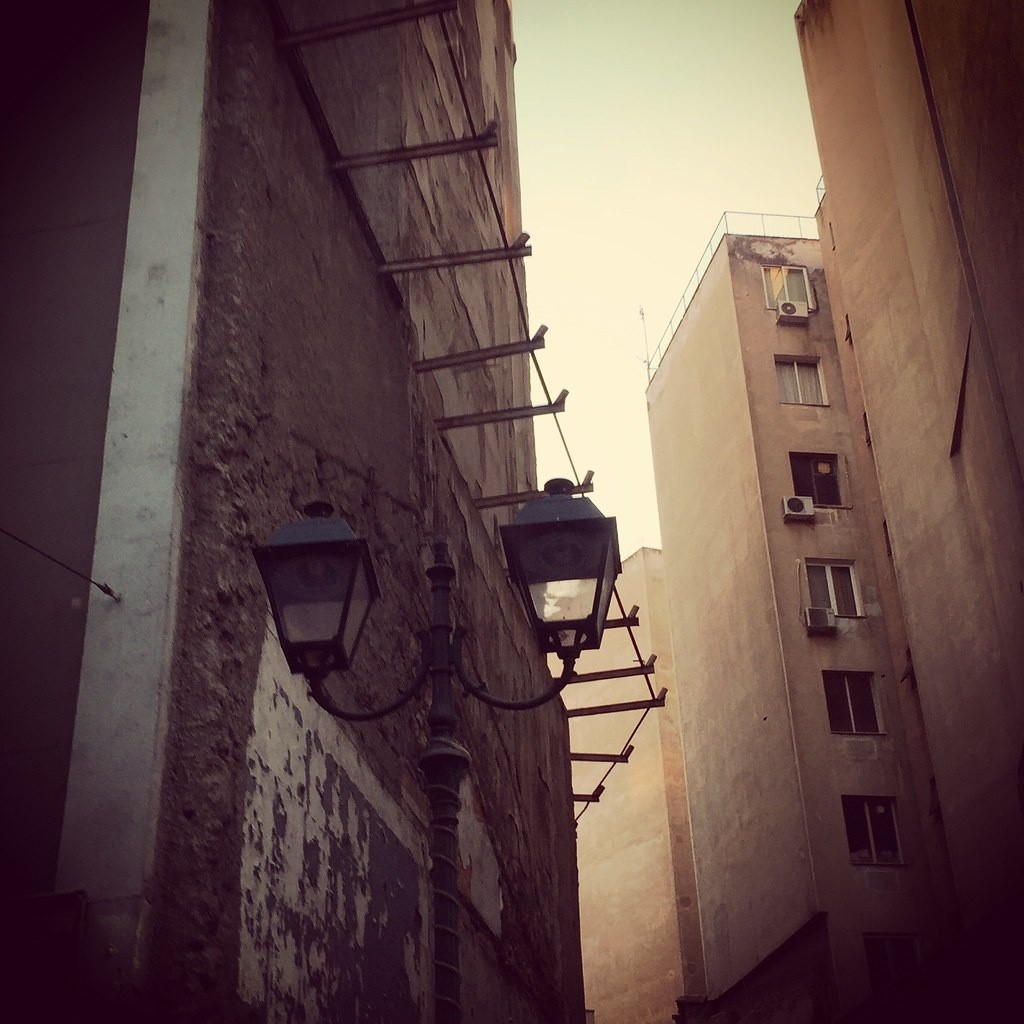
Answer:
[805,606,837,634]
[782,495,815,521]
[775,299,808,323]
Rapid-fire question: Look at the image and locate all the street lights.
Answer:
[247,479,623,1024]
